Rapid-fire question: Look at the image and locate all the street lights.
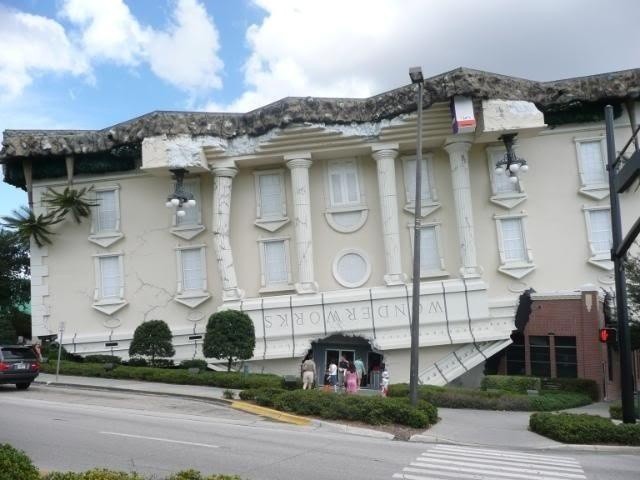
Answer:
[408,67,425,400]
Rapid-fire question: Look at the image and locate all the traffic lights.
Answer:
[600,328,618,344]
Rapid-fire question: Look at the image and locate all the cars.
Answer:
[0,343,40,390]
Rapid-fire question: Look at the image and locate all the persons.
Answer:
[379,370,390,398]
[352,355,367,389]
[345,362,359,394]
[338,354,351,389]
[301,353,317,390]
[326,358,338,392]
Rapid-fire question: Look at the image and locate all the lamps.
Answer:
[495,133,529,184]
[164,169,196,217]
